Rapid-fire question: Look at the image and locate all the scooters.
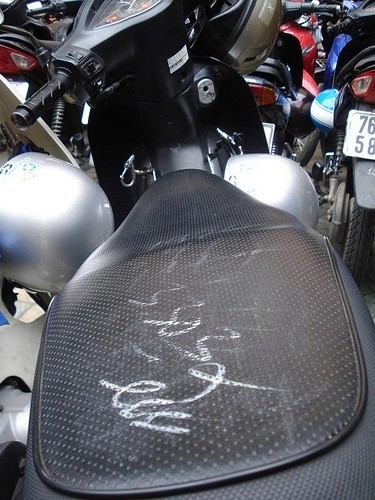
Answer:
[1,0,374,500]
[321,0,375,289]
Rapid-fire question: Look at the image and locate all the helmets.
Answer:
[0,151,114,298]
[206,0,282,77]
[310,89,339,133]
[224,153,319,230]
